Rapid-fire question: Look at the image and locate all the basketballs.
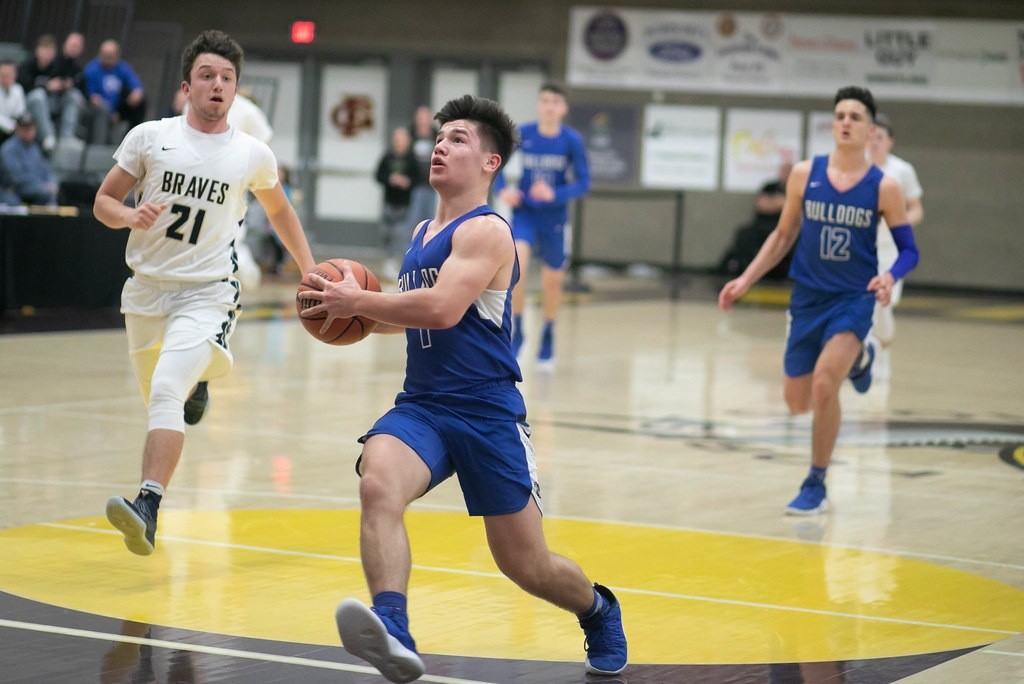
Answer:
[296,258,382,346]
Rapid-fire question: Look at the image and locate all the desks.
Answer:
[0,209,133,334]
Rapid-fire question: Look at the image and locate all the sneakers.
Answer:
[106,488,159,556]
[579,582,628,675]
[786,475,828,514]
[184,381,208,424]
[851,336,880,393]
[336,599,424,683]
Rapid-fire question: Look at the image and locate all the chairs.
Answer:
[0,42,128,172]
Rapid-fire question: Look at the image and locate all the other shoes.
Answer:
[539,334,552,360]
[511,333,523,357]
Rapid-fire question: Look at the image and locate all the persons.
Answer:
[863,112,926,350]
[297,93,627,684]
[701,180,798,288]
[374,103,439,280]
[82,38,146,143]
[261,166,292,273]
[93,28,318,557]
[491,85,592,368]
[178,77,273,288]
[18,30,110,155]
[0,59,63,206]
[715,86,920,518]
